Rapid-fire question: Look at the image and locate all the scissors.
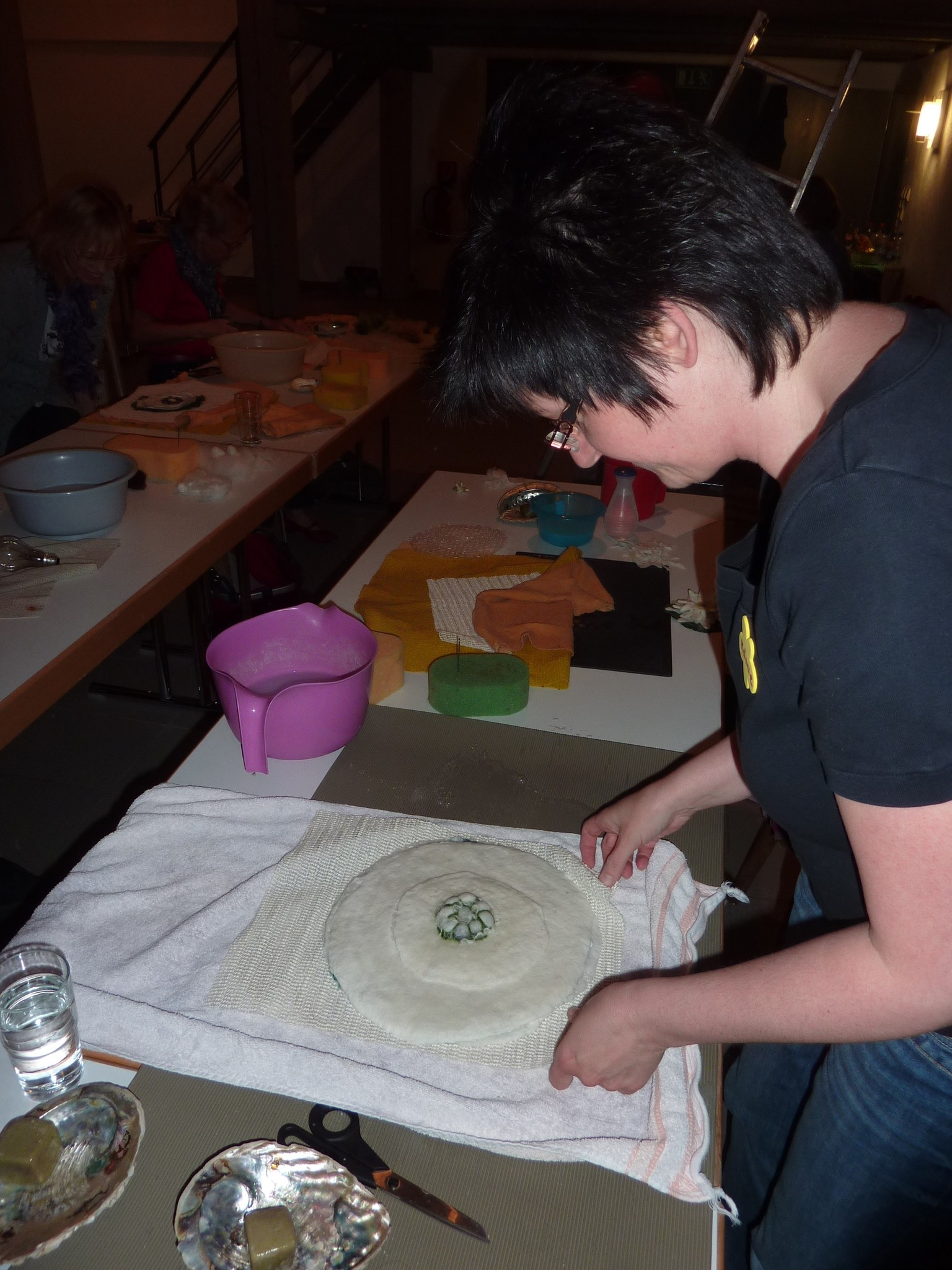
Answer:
[276,1102,494,1248]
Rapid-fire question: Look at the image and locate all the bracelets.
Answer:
[257,314,264,326]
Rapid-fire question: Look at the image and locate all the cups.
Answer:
[0,940,85,1102]
[234,391,263,445]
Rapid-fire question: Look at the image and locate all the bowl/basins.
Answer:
[532,490,605,547]
[3,446,140,542]
[204,600,378,775]
[209,330,311,386]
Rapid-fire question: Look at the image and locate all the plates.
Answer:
[1,1081,148,1270]
[173,1138,392,1270]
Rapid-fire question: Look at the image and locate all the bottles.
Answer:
[605,467,640,543]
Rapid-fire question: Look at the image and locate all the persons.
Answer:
[787,173,852,302]
[129,187,287,387]
[441,116,952,1270]
[1,181,134,459]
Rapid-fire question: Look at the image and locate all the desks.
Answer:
[0,465,738,1270]
[0,424,432,723]
[68,313,443,608]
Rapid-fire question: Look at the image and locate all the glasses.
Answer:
[543,385,590,453]
